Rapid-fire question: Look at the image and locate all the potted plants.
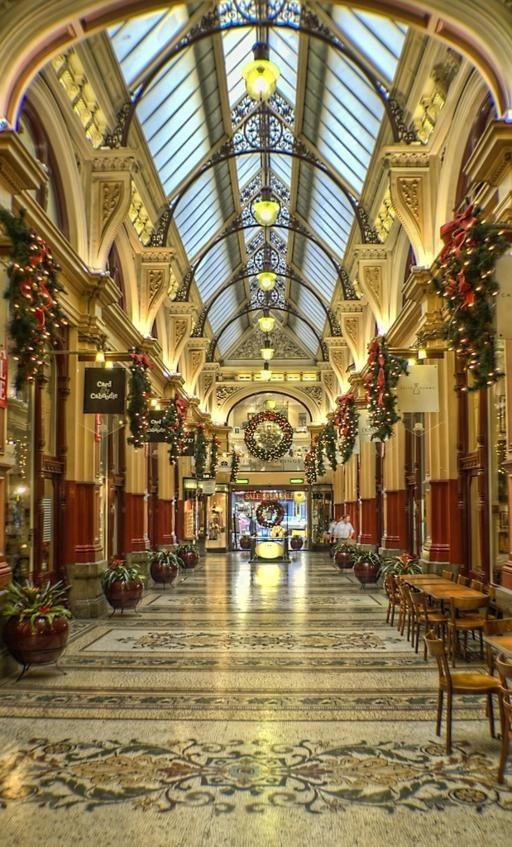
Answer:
[1,572,73,684]
[331,541,360,570]
[237,533,256,548]
[290,535,303,550]
[175,544,201,570]
[146,551,185,592]
[102,556,145,618]
[353,549,382,585]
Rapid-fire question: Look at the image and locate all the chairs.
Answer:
[383,568,512,784]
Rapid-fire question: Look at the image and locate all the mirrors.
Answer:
[489,340,512,597]
[6,353,33,563]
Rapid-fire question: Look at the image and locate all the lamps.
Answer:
[103,356,113,370]
[242,1,280,382]
[91,336,106,362]
[416,337,427,362]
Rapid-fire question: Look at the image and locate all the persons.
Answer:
[328,513,355,558]
